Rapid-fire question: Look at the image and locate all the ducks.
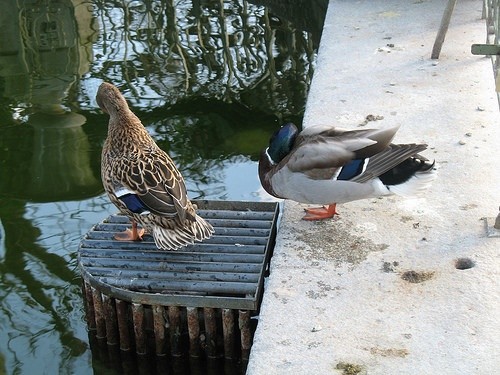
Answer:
[96,82,215,250]
[258,122,436,220]
